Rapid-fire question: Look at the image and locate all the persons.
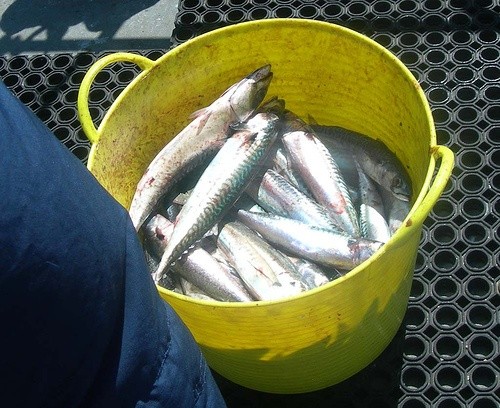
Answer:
[1,75,231,408]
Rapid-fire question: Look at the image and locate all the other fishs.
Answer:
[127,61,417,304]
